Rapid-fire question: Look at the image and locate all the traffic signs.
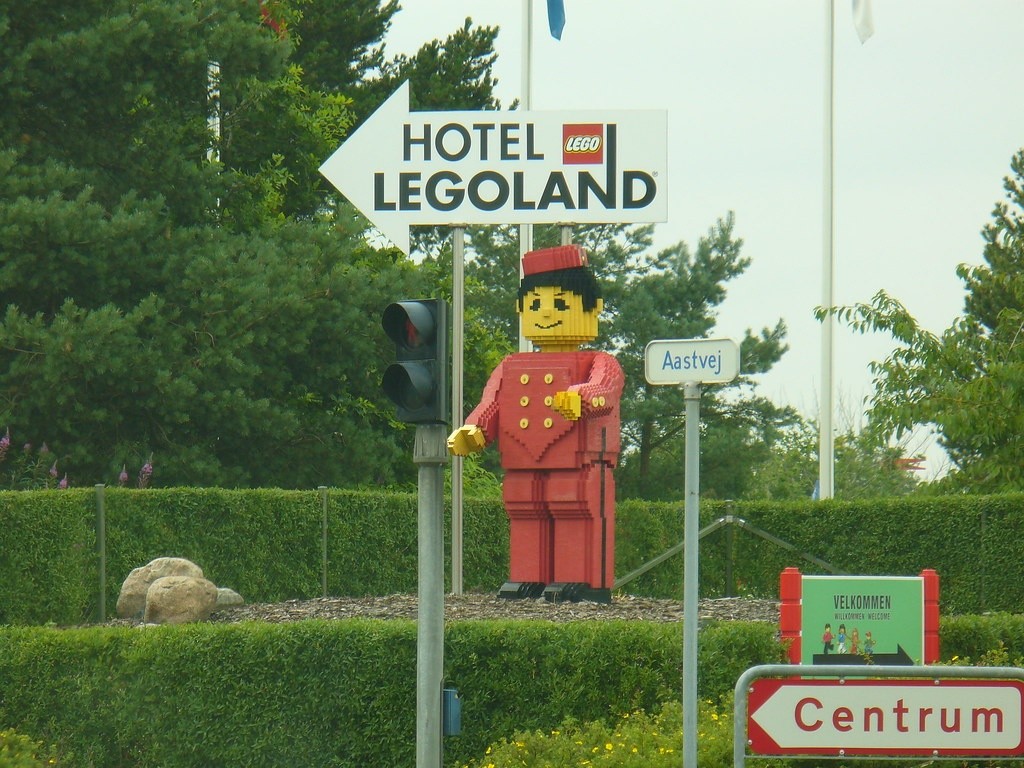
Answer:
[746,679,1024,760]
[317,79,669,259]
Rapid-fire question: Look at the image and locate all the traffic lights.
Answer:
[379,296,452,427]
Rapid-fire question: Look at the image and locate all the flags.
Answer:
[545,0,568,44]
[851,0,875,46]
[258,0,290,42]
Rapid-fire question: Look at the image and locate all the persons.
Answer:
[446,244,624,604]
